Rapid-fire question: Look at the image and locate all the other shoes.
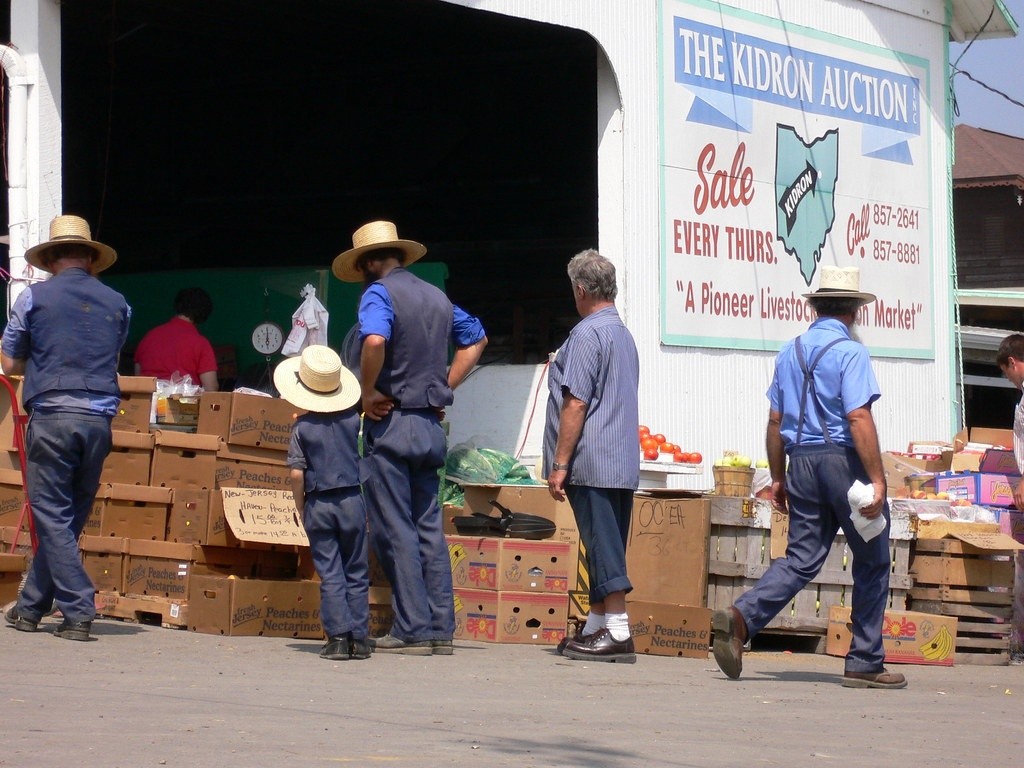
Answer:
[53,617,92,643]
[4,606,40,632]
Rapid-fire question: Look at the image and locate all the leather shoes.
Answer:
[557,625,596,654]
[712,605,747,678]
[842,664,907,689]
[431,639,453,655]
[318,632,349,659]
[347,637,370,659]
[367,632,433,656]
[562,627,636,664]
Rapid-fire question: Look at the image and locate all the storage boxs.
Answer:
[824,605,957,667]
[937,472,1024,506]
[916,534,1015,666]
[0,374,396,638]
[439,485,585,651]
[623,600,712,659]
[954,424,1018,473]
[892,449,953,472]
[614,496,709,605]
[988,506,1024,542]
[709,495,913,638]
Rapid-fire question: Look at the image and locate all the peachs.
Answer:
[894,489,973,506]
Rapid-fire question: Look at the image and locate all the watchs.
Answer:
[553,462,569,470]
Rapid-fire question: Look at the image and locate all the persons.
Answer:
[132,287,219,392]
[541,252,637,663]
[274,346,371,657]
[332,220,487,655]
[0,216,134,640]
[998,334,1024,514]
[712,266,908,688]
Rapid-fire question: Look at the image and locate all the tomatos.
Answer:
[637,425,703,464]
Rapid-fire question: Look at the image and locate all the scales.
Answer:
[250,287,287,399]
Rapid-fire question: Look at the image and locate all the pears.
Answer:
[714,455,768,468]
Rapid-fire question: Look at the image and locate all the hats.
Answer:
[332,220,427,283]
[24,215,117,275]
[801,266,876,306]
[273,345,362,412]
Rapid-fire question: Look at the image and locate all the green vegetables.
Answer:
[443,445,541,506]
